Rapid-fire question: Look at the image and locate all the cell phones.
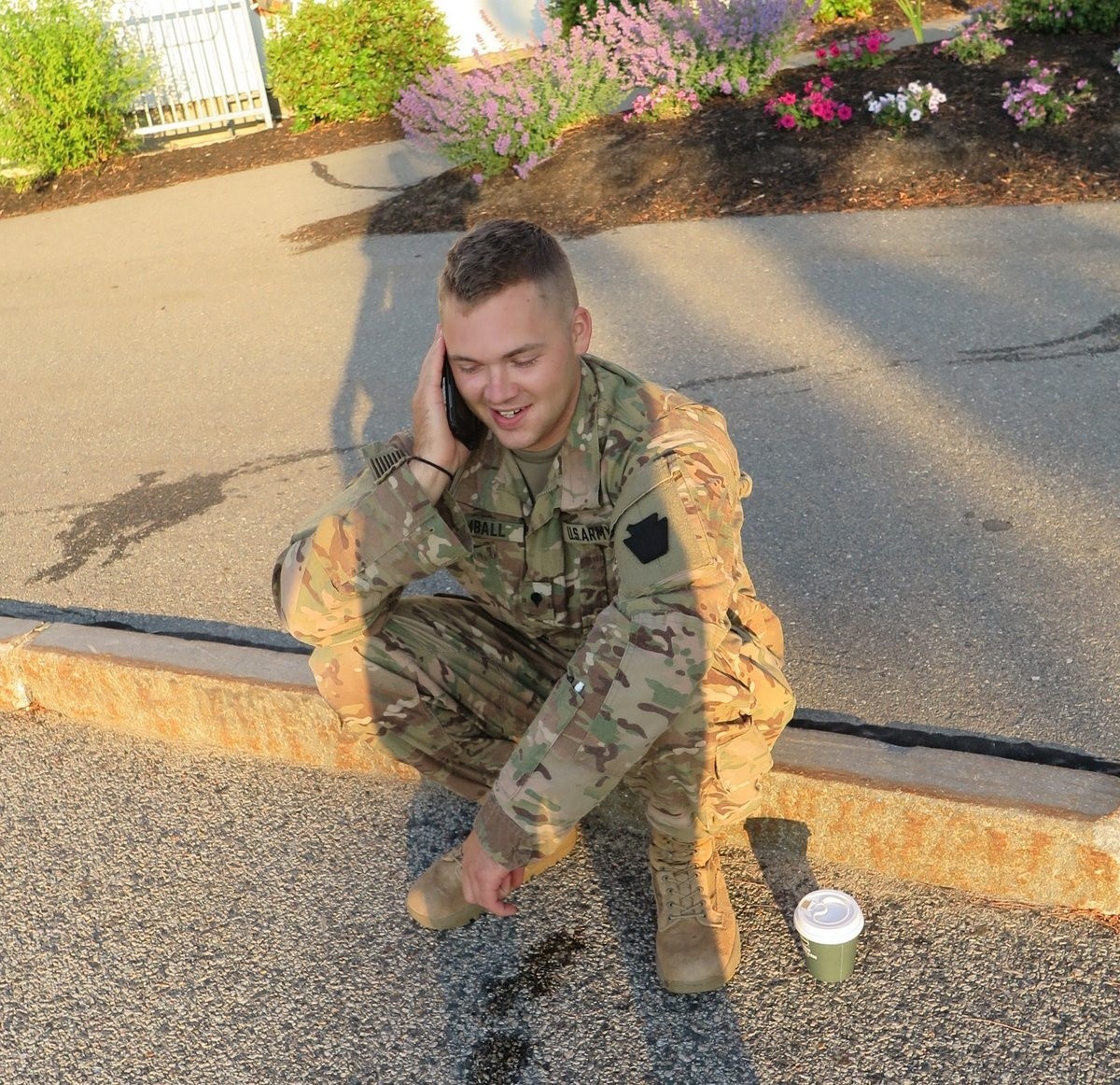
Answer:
[444,352,481,454]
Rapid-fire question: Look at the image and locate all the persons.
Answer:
[279,219,795,992]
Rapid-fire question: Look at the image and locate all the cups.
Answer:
[793,890,864,983]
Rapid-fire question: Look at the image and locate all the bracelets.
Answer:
[410,455,453,480]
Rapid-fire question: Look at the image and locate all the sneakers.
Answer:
[407,817,578,931]
[647,832,741,989]
